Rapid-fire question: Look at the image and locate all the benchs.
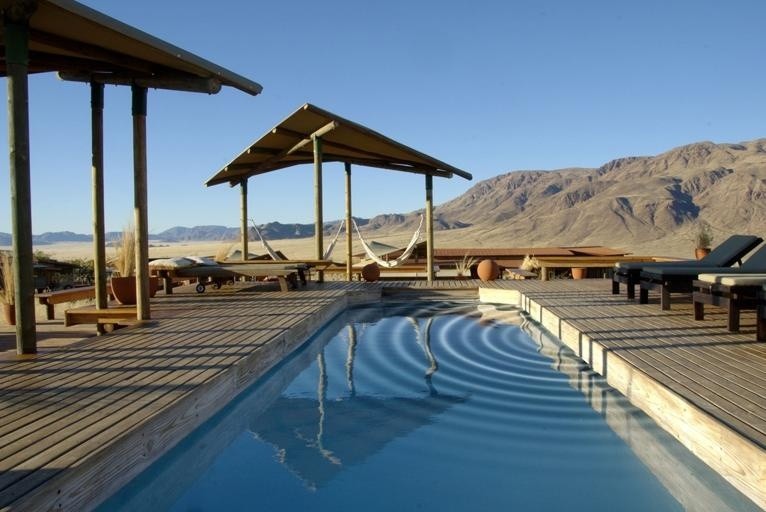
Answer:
[530,255,657,282]
[148,254,334,295]
[35,283,112,321]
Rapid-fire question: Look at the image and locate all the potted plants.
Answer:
[0,251,16,326]
[688,218,716,260]
[108,219,159,306]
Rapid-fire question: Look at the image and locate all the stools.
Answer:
[692,270,765,344]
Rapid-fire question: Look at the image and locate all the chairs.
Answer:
[611,234,765,299]
[638,243,765,311]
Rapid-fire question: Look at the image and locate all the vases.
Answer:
[570,266,588,279]
